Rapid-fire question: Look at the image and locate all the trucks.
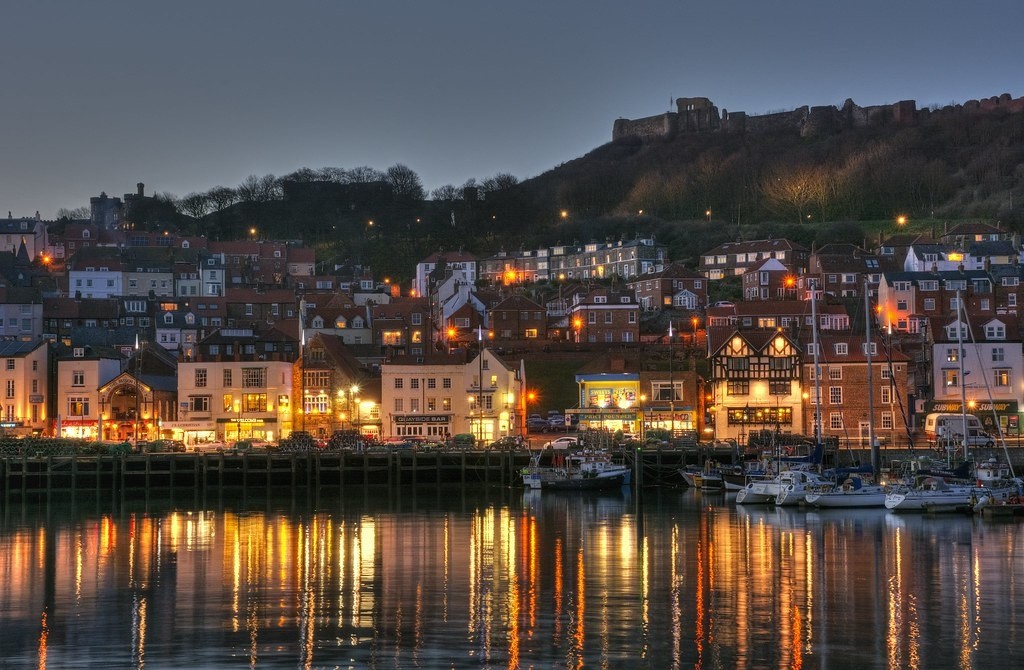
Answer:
[953,428,996,448]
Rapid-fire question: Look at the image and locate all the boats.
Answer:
[520,439,631,489]
[678,441,814,492]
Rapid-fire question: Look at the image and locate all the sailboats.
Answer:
[735,275,1024,513]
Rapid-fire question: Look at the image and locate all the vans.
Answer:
[925,413,984,445]
[548,410,559,419]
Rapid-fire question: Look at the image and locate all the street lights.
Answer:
[134,334,139,452]
[888,316,894,448]
[527,393,534,436]
[300,330,306,435]
[641,395,646,439]
[668,322,674,439]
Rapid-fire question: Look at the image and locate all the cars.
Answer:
[526,414,579,432]
[242,437,278,448]
[192,439,230,452]
[313,438,329,447]
[543,436,583,449]
[149,439,185,452]
[621,434,639,442]
[489,436,527,449]
